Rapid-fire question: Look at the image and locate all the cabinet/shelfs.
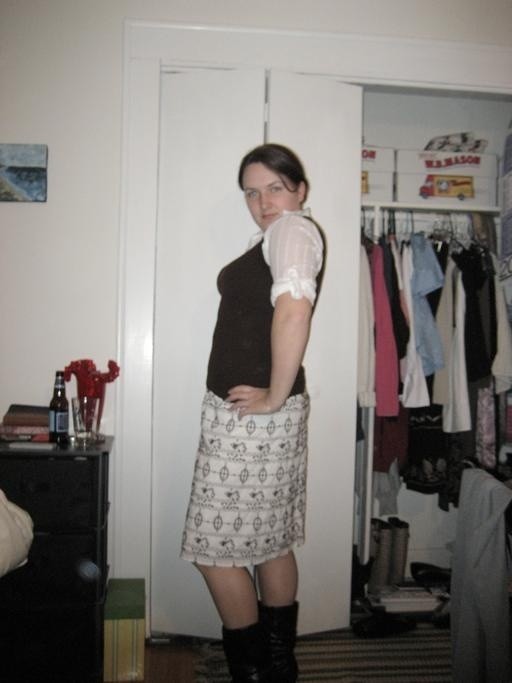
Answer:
[1,432,115,680]
[142,62,512,647]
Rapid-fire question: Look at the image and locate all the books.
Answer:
[9,441,58,450]
[1,431,50,442]
[3,403,50,423]
[1,423,50,434]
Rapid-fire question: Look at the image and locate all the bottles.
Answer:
[48,370,69,450]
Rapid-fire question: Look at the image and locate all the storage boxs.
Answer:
[359,145,500,207]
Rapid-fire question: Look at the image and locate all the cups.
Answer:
[71,397,101,450]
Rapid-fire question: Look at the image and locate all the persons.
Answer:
[179,141,328,683]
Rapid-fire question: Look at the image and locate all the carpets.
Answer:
[190,625,454,681]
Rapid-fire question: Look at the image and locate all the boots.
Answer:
[387,516,412,589]
[220,608,263,683]
[364,517,392,589]
[257,598,300,683]
